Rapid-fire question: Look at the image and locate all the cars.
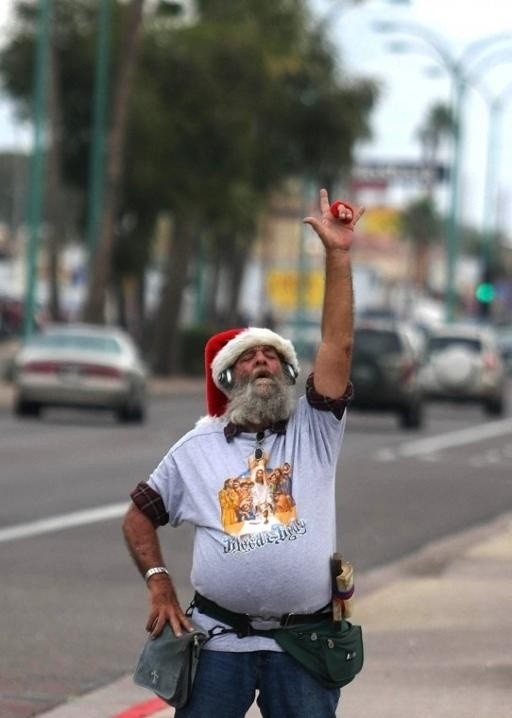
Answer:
[15,323,145,422]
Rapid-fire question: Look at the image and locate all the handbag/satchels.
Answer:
[133,622,210,709]
[271,621,363,689]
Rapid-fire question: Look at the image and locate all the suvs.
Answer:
[418,327,504,414]
[350,326,425,427]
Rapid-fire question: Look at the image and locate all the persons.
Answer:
[122,187,366,718]
[218,452,296,534]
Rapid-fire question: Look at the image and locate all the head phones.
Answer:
[218,362,299,387]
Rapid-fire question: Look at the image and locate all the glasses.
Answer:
[255,432,265,461]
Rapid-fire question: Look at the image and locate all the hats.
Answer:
[204,327,299,416]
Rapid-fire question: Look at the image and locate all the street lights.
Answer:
[376,20,509,317]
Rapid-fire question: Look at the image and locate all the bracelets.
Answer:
[144,566,170,583]
